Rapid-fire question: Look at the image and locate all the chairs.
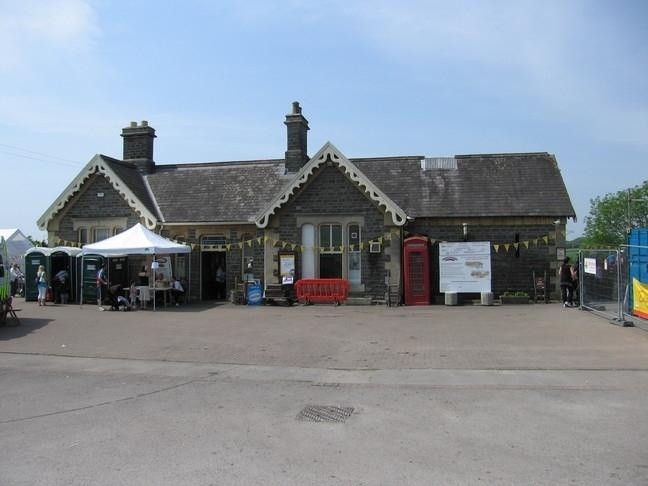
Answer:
[2,294,22,325]
[102,276,188,311]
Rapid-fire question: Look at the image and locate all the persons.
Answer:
[34,264,48,307]
[214,261,227,300]
[50,264,70,304]
[108,271,186,311]
[558,250,626,309]
[10,263,24,297]
[95,261,111,310]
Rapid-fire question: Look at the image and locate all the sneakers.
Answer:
[563,300,578,307]
[38,301,46,306]
[98,306,105,311]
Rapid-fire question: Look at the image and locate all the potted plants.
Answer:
[500,292,530,304]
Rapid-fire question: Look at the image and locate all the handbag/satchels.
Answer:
[570,267,578,280]
[35,277,39,283]
